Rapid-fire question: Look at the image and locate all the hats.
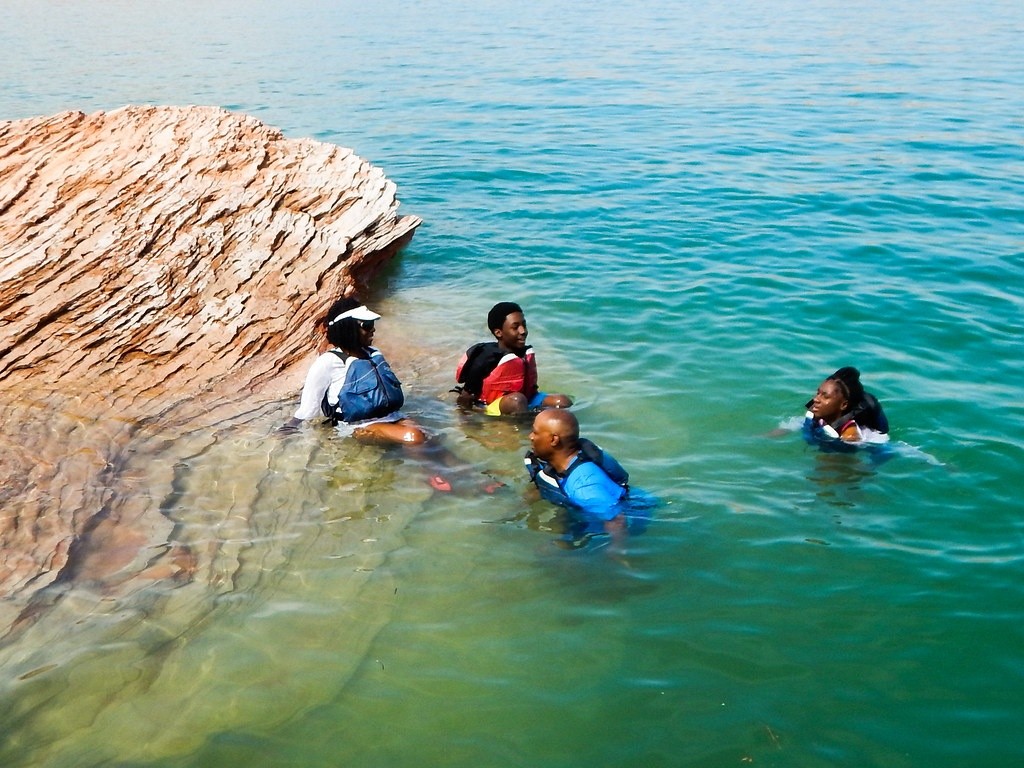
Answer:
[329,305,381,325]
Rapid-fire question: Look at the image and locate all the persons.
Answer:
[449,302,574,452]
[518,408,665,568]
[756,365,890,485]
[273,299,508,495]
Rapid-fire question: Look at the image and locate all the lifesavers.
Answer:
[322,345,404,420]
[526,438,628,515]
[805,391,891,454]
[453,341,537,408]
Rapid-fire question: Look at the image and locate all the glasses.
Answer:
[357,320,374,331]
